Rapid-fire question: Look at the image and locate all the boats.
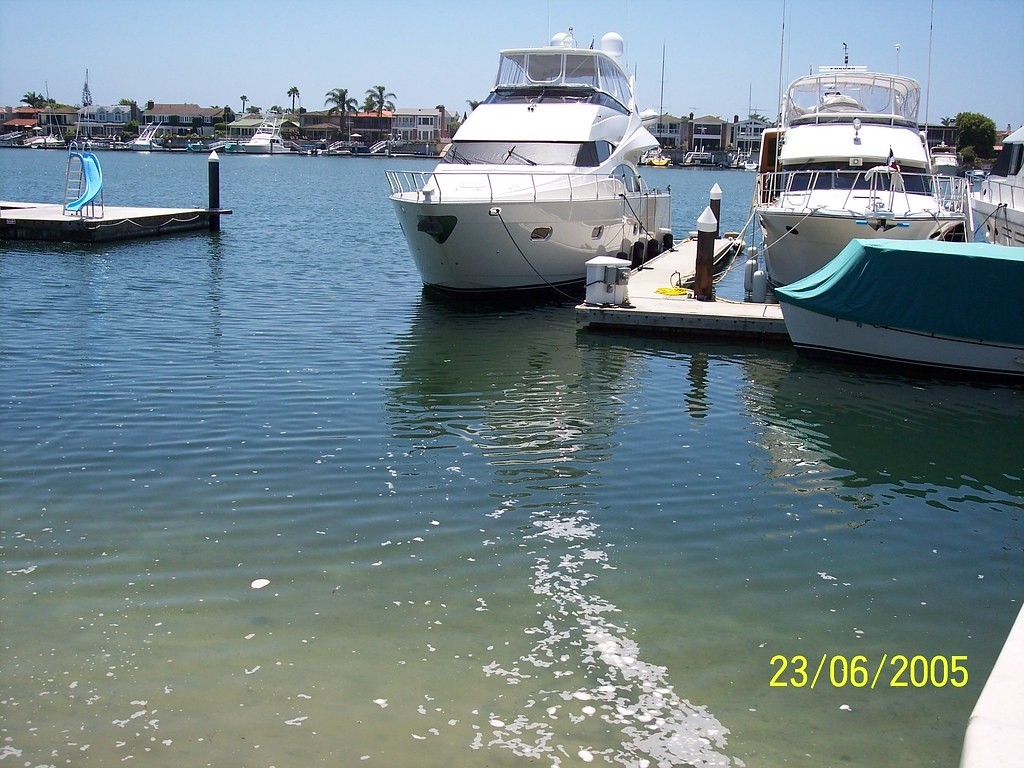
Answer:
[967,123,1024,246]
[637,146,758,170]
[0,80,302,156]
[930,145,959,179]
[771,237,1024,384]
[383,32,672,301]
[742,0,969,304]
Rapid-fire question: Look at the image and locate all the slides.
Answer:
[66,152,102,212]
